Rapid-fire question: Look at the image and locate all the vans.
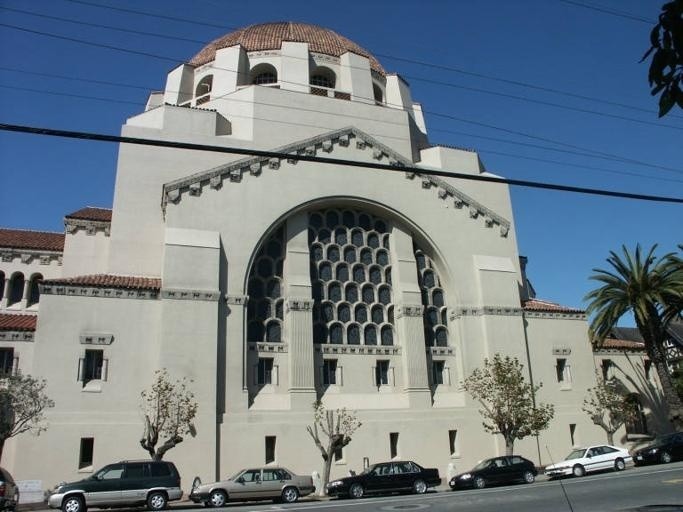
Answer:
[43,459,183,512]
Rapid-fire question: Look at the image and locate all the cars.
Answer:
[325,461,441,498]
[544,444,633,478]
[189,466,316,507]
[0,467,20,511]
[633,431,683,466]
[448,455,537,488]
